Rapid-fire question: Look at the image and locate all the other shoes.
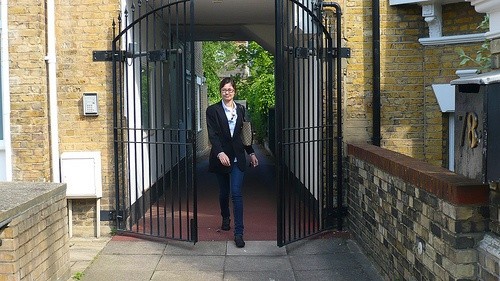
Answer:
[234,235,245,247]
[221,216,230,230]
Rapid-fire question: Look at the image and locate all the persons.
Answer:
[205,76,260,248]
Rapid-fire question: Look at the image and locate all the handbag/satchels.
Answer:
[238,103,253,146]
